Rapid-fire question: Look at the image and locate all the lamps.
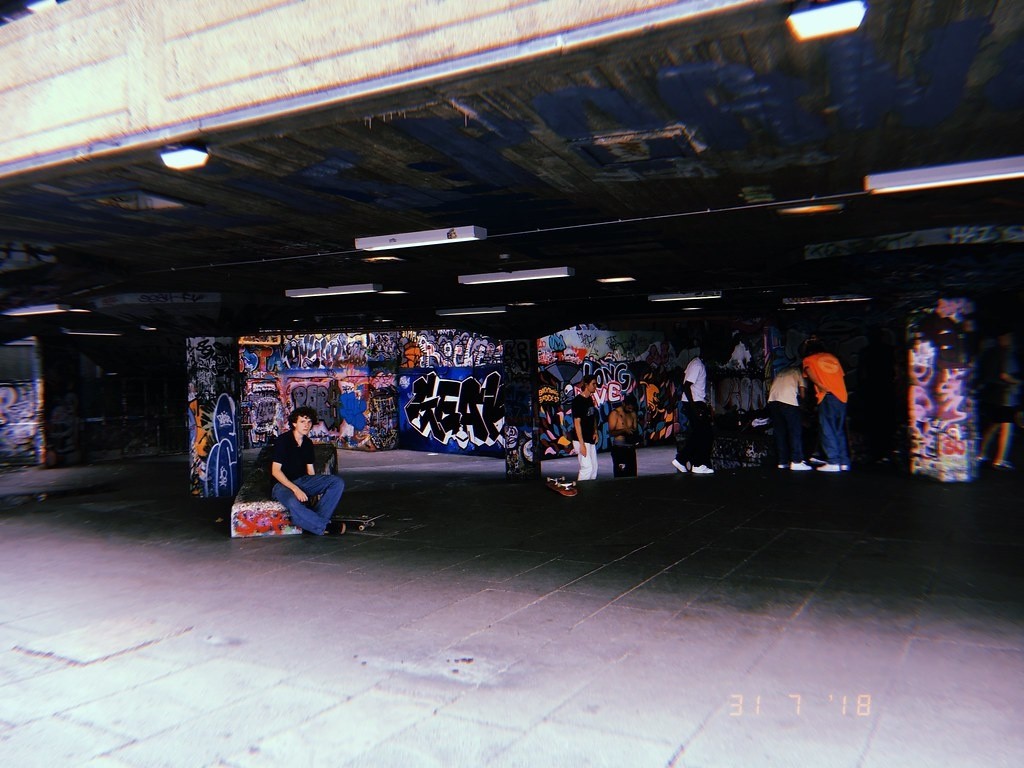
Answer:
[355,226,488,251]
[458,267,577,285]
[439,305,510,316]
[787,0,868,42]
[649,290,723,302]
[160,138,209,171]
[863,156,1024,194]
[285,283,382,297]
[3,304,69,316]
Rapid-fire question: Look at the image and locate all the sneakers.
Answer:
[778,464,789,468]
[324,523,346,534]
[672,459,688,472]
[790,462,812,470]
[817,464,848,471]
[692,465,714,473]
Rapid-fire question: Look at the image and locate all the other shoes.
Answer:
[877,457,890,464]
[992,461,1013,469]
[802,456,830,464]
[976,456,992,462]
[892,448,907,455]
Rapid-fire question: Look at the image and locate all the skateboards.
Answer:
[545,476,578,498]
[331,512,384,530]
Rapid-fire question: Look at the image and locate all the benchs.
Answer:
[231,444,339,538]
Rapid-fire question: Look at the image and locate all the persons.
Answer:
[799,336,851,471]
[671,346,718,473]
[974,328,1021,468]
[766,364,813,470]
[571,374,600,481]
[607,396,639,477]
[263,406,347,536]
